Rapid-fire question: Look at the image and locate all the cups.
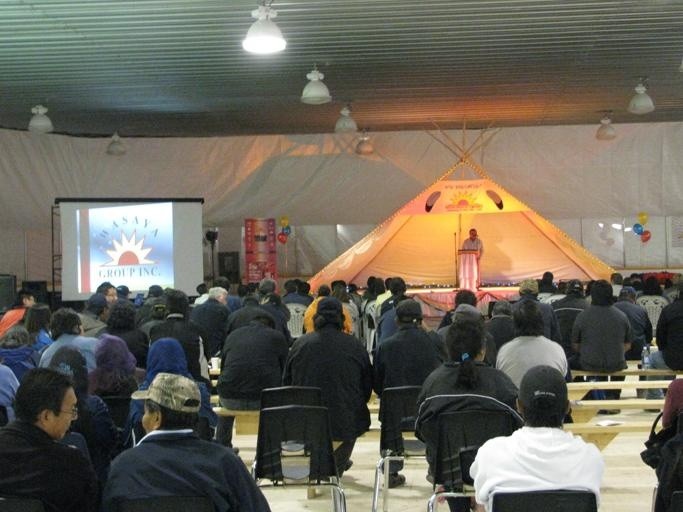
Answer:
[210,357,219,368]
[649,346,659,353]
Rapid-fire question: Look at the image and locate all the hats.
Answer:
[116,284,132,294]
[518,277,540,296]
[131,373,203,415]
[315,297,344,314]
[566,279,586,294]
[618,286,639,295]
[517,362,567,414]
[394,300,424,324]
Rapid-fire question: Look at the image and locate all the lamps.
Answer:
[26,104,53,135]
[241,0,286,55]
[353,126,373,155]
[105,129,126,157]
[333,102,357,134]
[625,73,656,115]
[300,62,333,105]
[596,110,617,141]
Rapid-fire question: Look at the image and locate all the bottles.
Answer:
[641,345,647,368]
[650,337,656,345]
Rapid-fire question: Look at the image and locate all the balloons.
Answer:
[282,226,291,235]
[633,223,644,235]
[278,232,288,245]
[638,212,648,225]
[280,216,289,227]
[641,230,651,242]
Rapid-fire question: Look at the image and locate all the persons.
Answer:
[463,229,484,288]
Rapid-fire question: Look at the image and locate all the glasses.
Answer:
[57,407,78,417]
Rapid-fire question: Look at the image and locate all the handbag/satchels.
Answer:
[641,413,677,467]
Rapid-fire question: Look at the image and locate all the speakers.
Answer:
[22,281,46,304]
[0,275,16,312]
[219,252,239,284]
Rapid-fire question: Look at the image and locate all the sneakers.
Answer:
[383,471,406,491]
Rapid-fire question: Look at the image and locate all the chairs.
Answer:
[2,494,47,512]
[62,431,91,457]
[367,384,597,511]
[249,384,346,512]
[106,494,216,512]
[343,298,382,366]
[285,302,308,339]
[100,395,134,429]
[634,288,679,357]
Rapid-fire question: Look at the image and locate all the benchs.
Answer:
[210,368,259,435]
[562,360,679,450]
